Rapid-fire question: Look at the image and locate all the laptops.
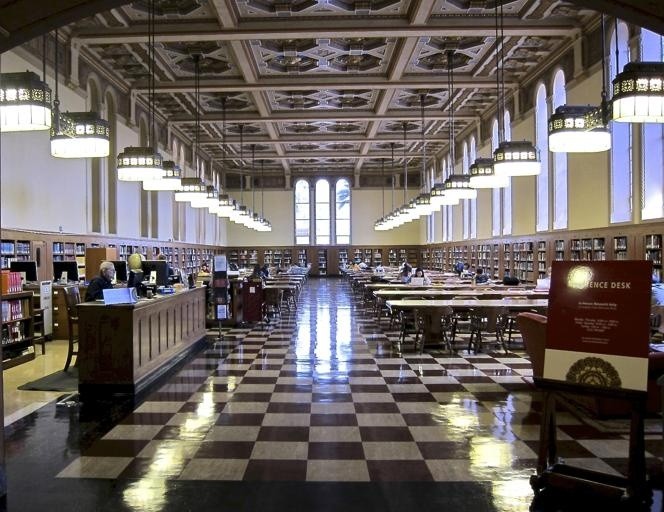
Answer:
[409,277,424,286]
[533,278,551,292]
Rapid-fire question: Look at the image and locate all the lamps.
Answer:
[610,3,663,124]
[547,2,612,155]
[467,2,510,187]
[1,1,50,130]
[49,2,112,160]
[441,46,478,201]
[116,1,162,182]
[374,92,442,231]
[142,4,182,190]
[493,2,541,177]
[174,44,272,233]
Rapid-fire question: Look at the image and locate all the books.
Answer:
[421,235,664,284]
[0,243,229,363]
[229,249,418,274]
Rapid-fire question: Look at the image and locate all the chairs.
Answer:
[63,285,82,372]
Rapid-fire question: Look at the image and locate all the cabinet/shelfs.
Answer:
[0,227,226,370]
[226,244,425,278]
[425,218,664,288]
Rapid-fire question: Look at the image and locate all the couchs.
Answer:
[515,310,664,421]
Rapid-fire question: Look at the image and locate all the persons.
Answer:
[406,268,431,284]
[251,262,274,286]
[651,273,664,328]
[260,263,273,279]
[397,267,410,281]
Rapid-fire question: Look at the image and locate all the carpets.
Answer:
[16,366,79,393]
[522,376,664,435]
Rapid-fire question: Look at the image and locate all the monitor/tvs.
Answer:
[53,261,79,287]
[10,261,37,286]
[100,260,127,284]
[127,269,144,296]
[141,260,168,285]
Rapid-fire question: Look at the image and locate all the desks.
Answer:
[193,269,311,316]
[338,265,551,355]
